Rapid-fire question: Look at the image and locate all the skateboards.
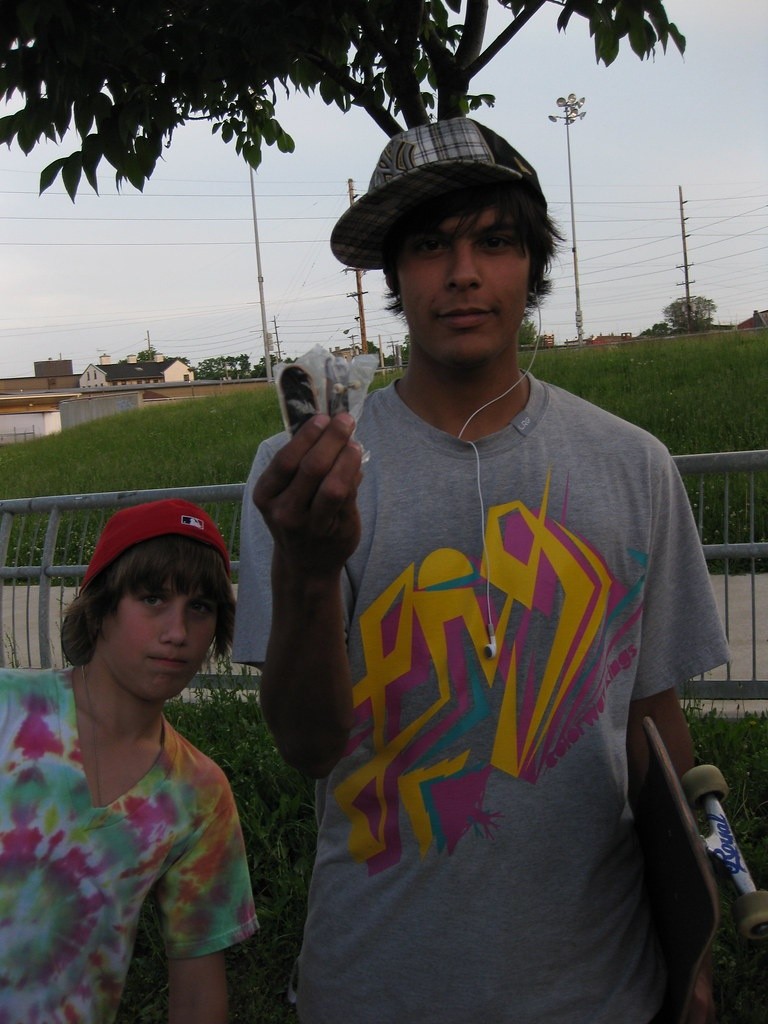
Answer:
[639,715,768,1024]
[278,364,320,441]
[324,354,362,421]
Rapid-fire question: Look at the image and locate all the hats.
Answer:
[60,498,232,667]
[331,119,547,269]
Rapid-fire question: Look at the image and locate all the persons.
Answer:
[0,498,262,1024]
[227,115,732,1024]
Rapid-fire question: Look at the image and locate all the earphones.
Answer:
[484,623,497,659]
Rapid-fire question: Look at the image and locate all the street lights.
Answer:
[548,94,588,341]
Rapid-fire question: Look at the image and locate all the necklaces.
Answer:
[81,665,102,808]
